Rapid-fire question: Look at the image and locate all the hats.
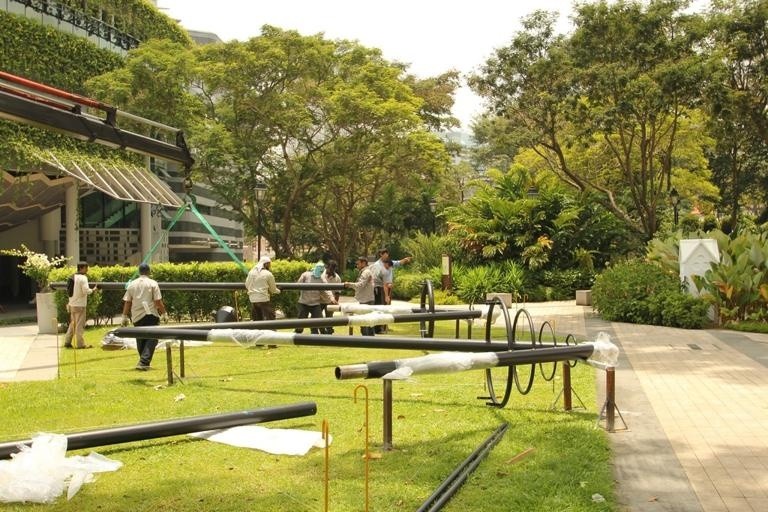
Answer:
[314,263,325,278]
[139,264,150,273]
[356,256,368,263]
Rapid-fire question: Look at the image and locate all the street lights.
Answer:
[670,188,681,226]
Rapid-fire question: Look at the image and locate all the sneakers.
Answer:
[65,343,92,348]
[136,361,151,371]
[256,344,278,348]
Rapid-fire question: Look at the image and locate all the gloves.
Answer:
[121,315,130,327]
[160,313,169,324]
[401,257,413,264]
[344,281,353,288]
[275,289,280,294]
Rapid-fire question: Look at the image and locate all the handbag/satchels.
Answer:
[67,277,74,297]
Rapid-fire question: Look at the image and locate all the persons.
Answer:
[293,248,415,336]
[121,262,169,370]
[63,261,98,349]
[245,255,281,348]
